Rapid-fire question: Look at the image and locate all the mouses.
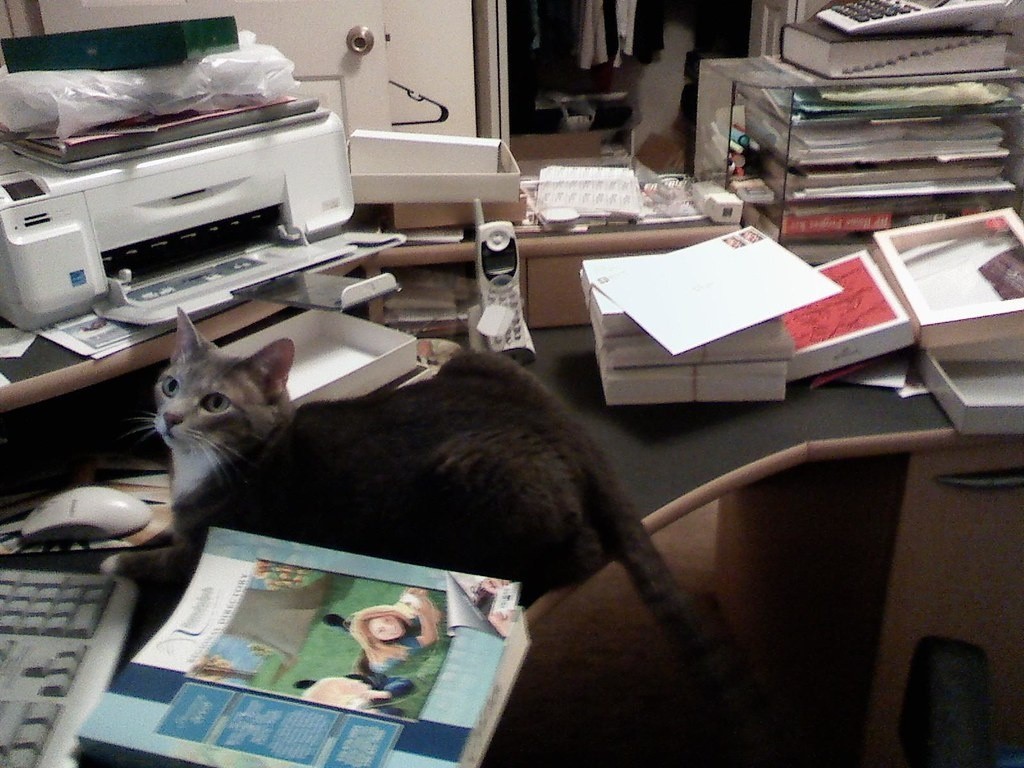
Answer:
[21,486,154,541]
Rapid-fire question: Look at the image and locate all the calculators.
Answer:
[816,0,1020,36]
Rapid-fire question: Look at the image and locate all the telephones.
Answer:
[467,197,536,367]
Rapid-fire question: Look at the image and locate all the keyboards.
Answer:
[0,568,139,768]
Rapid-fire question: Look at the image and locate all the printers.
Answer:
[0,100,407,331]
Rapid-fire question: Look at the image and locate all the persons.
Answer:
[290,588,443,716]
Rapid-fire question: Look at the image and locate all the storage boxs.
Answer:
[778,250,916,383]
[345,128,521,207]
[909,343,1024,436]
[873,207,1024,348]
[389,185,530,230]
[217,308,419,411]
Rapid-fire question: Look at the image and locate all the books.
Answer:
[74,527,531,767]
[10,93,320,163]
[784,249,916,380]
[579,256,796,405]
[766,158,1006,185]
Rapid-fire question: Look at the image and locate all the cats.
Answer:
[97,305,708,662]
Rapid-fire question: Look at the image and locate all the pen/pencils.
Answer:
[700,121,761,176]
